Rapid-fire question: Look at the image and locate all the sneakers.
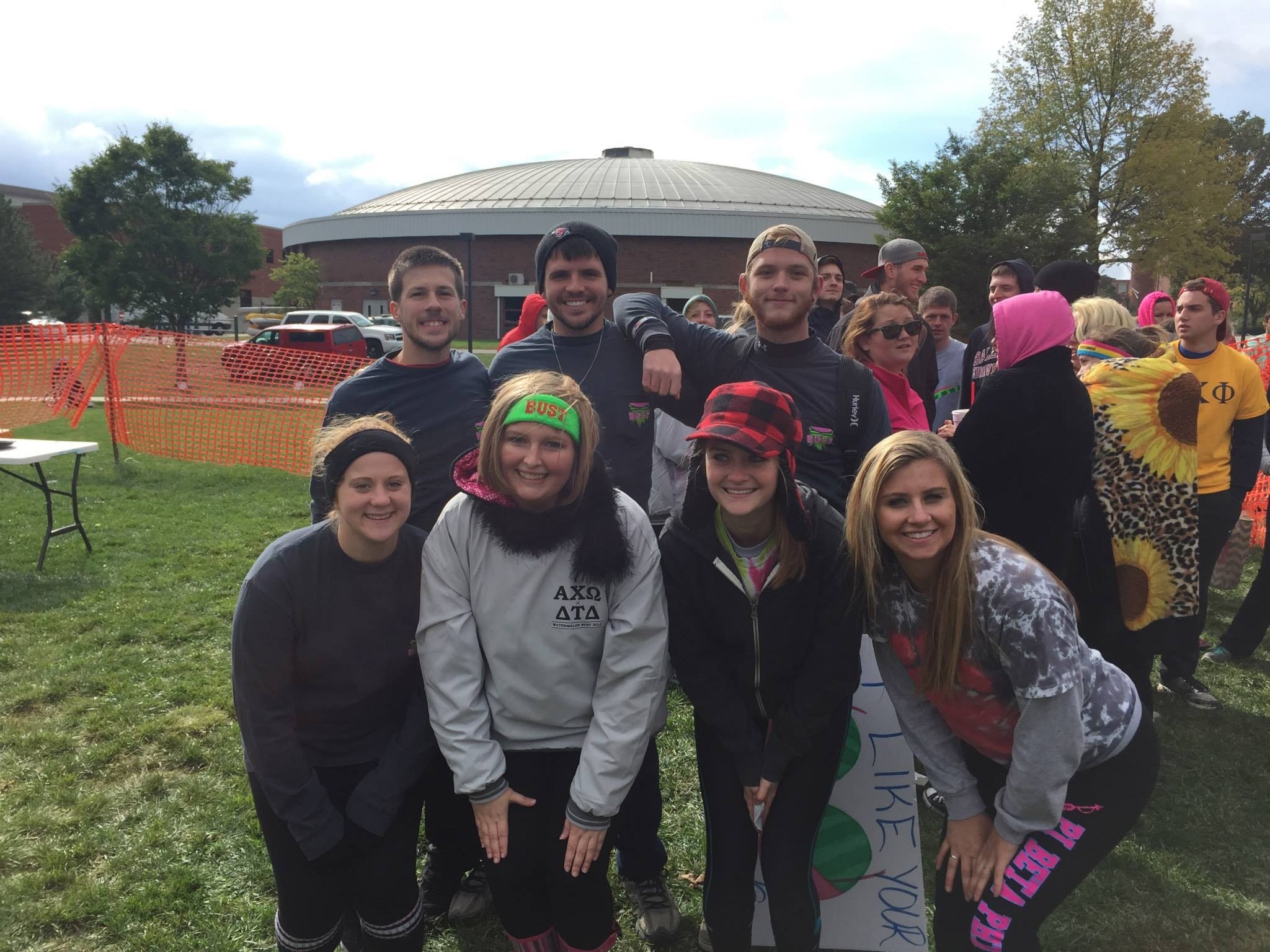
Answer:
[1157,674,1220,709]
[448,865,492,928]
[619,868,680,944]
[923,788,945,817]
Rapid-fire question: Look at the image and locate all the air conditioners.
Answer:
[508,272,526,286]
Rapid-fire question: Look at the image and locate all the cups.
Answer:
[951,409,970,433]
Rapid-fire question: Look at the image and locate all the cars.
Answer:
[20,310,68,342]
[367,313,401,327]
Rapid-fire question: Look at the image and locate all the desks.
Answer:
[0,435,100,574]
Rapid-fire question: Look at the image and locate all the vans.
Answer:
[119,295,234,336]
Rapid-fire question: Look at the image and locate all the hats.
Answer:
[746,224,818,273]
[861,238,928,279]
[685,381,813,528]
[1177,278,1231,342]
[535,220,618,298]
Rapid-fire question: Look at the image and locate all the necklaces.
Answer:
[551,326,604,386]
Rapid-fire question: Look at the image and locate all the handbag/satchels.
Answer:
[1211,515,1253,591]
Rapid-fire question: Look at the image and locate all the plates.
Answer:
[0,441,13,449]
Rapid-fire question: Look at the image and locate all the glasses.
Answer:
[867,320,923,340]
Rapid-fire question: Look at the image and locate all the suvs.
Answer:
[221,322,368,385]
[281,310,403,361]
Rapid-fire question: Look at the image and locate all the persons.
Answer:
[497,293,548,353]
[658,381,866,952]
[416,371,668,952]
[231,412,436,952]
[648,239,1270,718]
[612,224,893,952]
[449,220,682,944]
[310,245,492,934]
[845,428,1160,952]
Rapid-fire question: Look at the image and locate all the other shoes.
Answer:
[1198,638,1211,647]
[420,879,458,915]
[1201,648,1244,668]
[698,918,713,952]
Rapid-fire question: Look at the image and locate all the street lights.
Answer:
[458,232,476,354]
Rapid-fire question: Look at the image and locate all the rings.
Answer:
[949,855,957,859]
